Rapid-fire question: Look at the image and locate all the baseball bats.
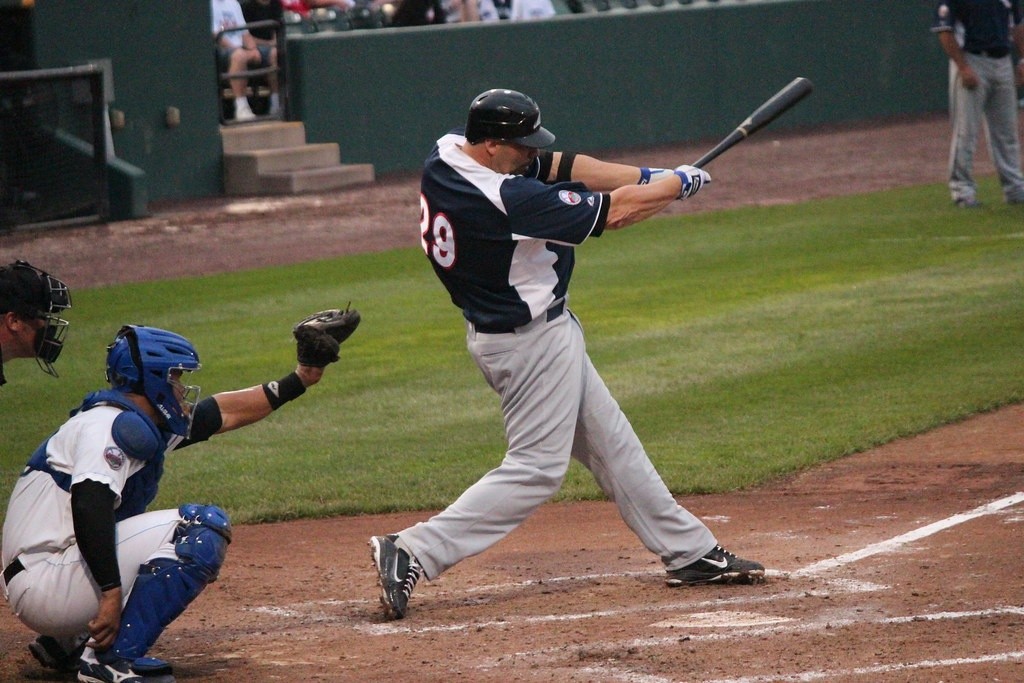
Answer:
[689,76,814,169]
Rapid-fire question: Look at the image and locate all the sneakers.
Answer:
[24,628,176,683]
[660,540,774,590]
[367,533,424,621]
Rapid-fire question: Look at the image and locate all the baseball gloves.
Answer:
[291,302,361,368]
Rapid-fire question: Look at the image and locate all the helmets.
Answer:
[0,258,51,318]
[467,88,558,149]
[103,324,206,440]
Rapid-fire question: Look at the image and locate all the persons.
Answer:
[0,307,361,683]
[0,259,73,390]
[368,88,766,619]
[212,0,556,123]
[929,0,1024,207]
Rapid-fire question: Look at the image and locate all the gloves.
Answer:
[637,154,720,204]
